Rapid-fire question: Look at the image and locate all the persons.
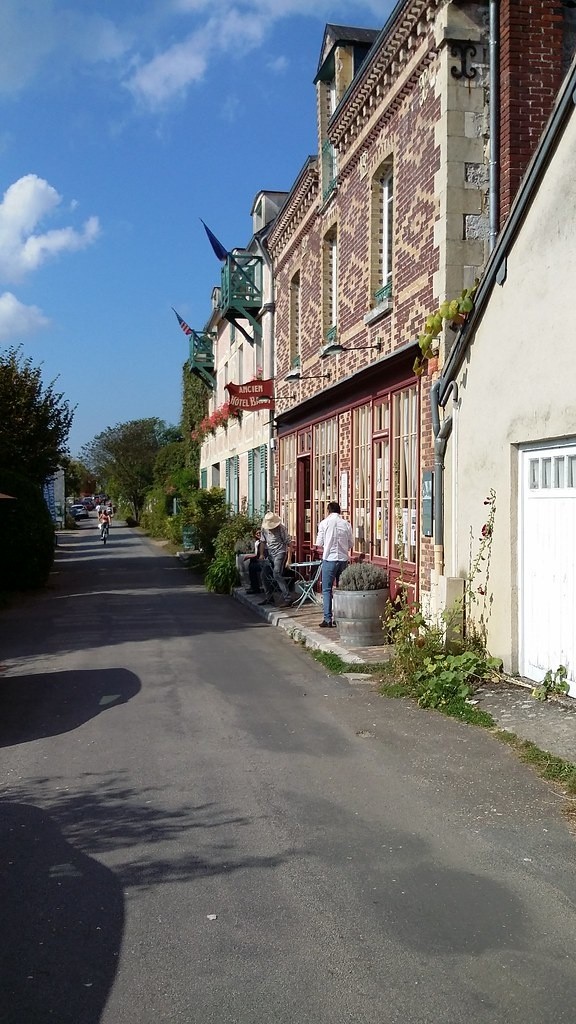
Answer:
[95,501,113,540]
[316,502,354,628]
[243,512,294,608]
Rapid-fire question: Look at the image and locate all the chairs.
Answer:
[256,559,294,606]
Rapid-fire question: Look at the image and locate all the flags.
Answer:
[171,306,195,335]
[199,216,230,261]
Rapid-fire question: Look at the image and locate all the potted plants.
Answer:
[334,563,391,645]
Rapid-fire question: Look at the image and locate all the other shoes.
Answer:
[281,601,293,606]
[259,597,275,605]
[245,586,260,594]
[319,620,332,627]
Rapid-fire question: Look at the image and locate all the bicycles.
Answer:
[101,524,108,545]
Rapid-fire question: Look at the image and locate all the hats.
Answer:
[262,513,281,530]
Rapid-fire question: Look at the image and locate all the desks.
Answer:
[288,561,324,614]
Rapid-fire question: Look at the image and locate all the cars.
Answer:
[69,504,89,519]
[79,493,116,519]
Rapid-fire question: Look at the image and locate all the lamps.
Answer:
[284,372,330,383]
[324,338,382,356]
[258,395,296,403]
